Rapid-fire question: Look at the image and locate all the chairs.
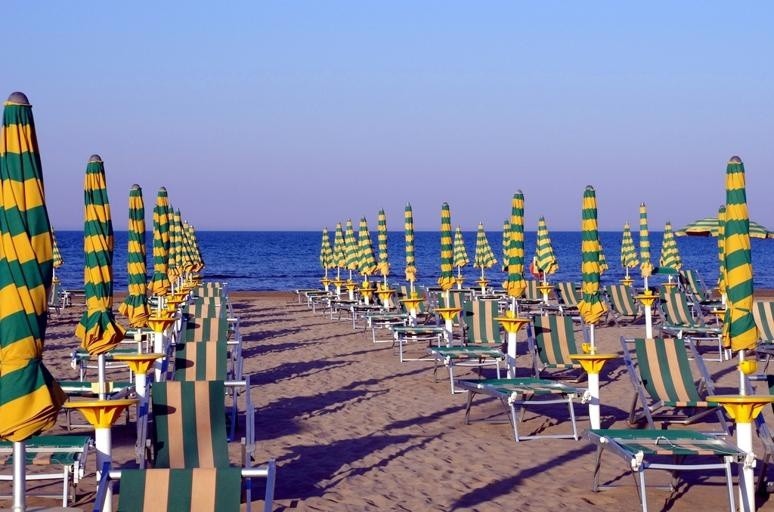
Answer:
[0,380,277,512]
[48,289,87,309]
[146,280,240,329]
[582,335,774,512]
[294,270,732,395]
[69,329,243,380]
[458,377,580,441]
[750,295,774,371]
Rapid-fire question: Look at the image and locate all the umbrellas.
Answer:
[150,183,202,320]
[73,154,128,401]
[118,181,153,355]
[0,92,68,511]
